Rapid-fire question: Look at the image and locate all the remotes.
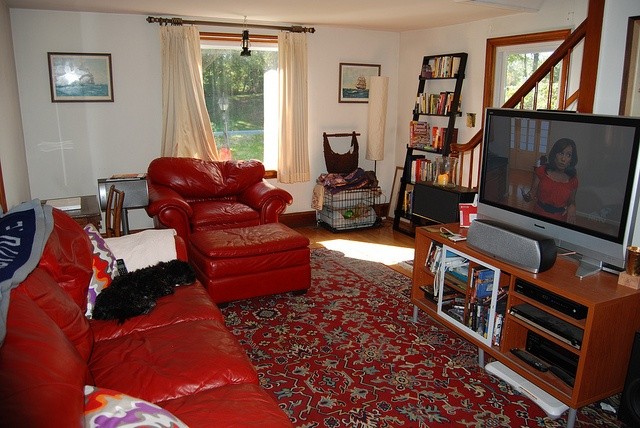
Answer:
[509,346,549,378]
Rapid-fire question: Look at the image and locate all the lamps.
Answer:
[364,67,388,217]
[240,16,252,58]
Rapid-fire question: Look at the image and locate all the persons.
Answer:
[524,138,579,224]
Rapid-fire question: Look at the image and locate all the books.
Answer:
[410,121,458,152]
[404,190,413,214]
[415,91,462,115]
[422,56,460,77]
[411,159,440,182]
[419,240,510,348]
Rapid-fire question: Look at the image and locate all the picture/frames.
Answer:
[48,53,115,102]
[340,62,382,104]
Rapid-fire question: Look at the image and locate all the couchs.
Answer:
[147,155,310,304]
[1,198,300,427]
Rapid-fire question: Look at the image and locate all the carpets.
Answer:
[210,239,637,427]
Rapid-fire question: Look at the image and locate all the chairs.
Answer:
[96,182,127,236]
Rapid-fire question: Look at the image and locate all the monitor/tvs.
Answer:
[475,107,640,279]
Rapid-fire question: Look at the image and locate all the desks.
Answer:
[23,195,102,235]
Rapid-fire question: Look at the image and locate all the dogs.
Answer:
[92,258,196,325]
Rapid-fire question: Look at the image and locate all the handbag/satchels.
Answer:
[323,130,359,173]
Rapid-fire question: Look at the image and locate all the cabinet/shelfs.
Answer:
[409,213,640,425]
[394,54,468,237]
